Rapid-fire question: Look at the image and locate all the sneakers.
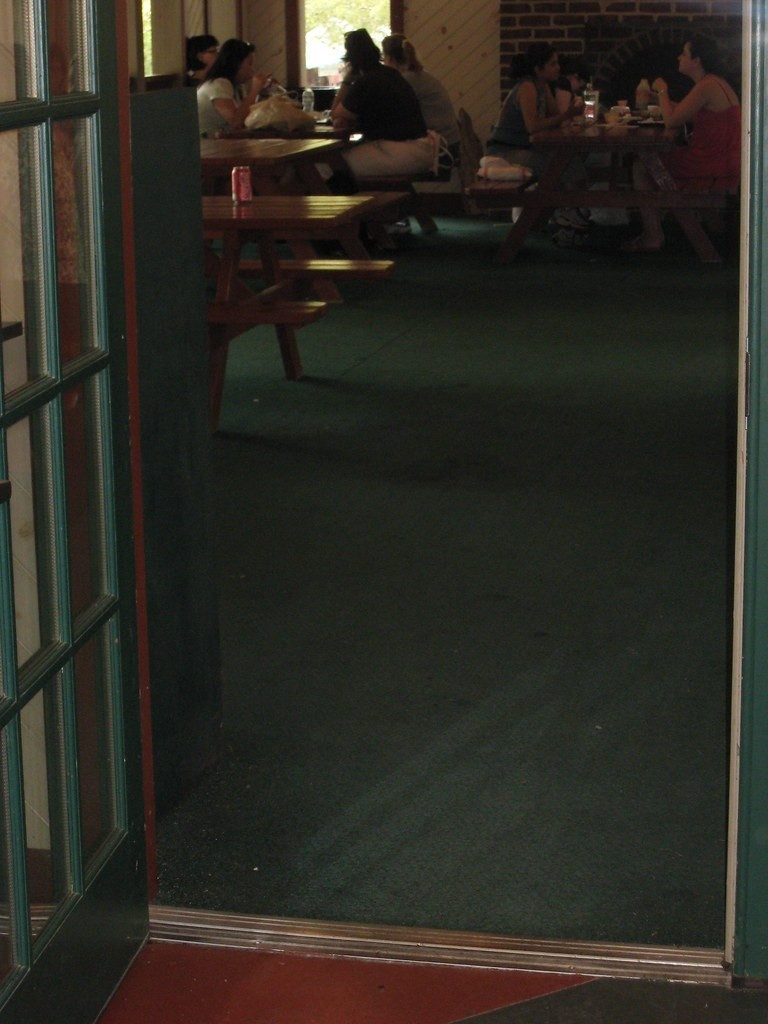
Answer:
[557,208,592,230]
[552,226,592,250]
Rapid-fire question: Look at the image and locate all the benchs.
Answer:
[468,191,737,260]
[165,168,453,426]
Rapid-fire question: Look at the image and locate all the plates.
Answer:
[637,120,665,124]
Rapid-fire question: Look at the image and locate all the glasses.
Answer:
[203,49,219,56]
[236,41,250,51]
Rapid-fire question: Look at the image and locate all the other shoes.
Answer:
[384,216,411,233]
[683,256,724,266]
[619,235,665,253]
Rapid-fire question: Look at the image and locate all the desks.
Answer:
[198,136,387,275]
[498,111,722,266]
[199,193,374,435]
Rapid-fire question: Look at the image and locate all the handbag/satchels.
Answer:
[426,129,454,176]
[477,156,533,180]
[244,91,315,134]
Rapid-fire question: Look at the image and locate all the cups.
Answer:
[648,106,658,121]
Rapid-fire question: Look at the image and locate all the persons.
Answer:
[494,29,741,251]
[185,29,461,258]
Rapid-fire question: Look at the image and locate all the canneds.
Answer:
[231,165,252,203]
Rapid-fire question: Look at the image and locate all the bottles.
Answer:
[583,82,595,119]
[634,78,651,110]
[302,86,314,112]
